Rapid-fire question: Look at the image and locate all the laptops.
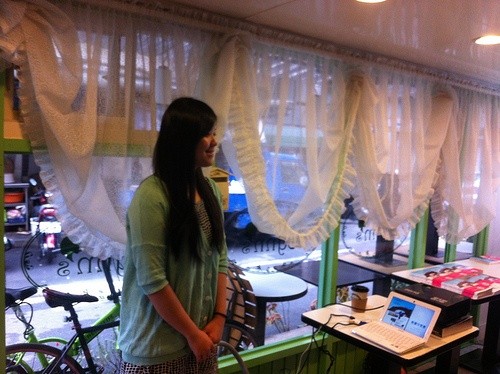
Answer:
[352,291,442,354]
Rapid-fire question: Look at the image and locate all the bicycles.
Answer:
[5,257,122,373]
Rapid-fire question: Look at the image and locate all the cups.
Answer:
[351,286,369,312]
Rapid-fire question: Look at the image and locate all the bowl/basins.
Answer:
[4,193,24,204]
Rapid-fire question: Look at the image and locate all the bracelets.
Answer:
[214,312,227,319]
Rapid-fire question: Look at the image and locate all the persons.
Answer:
[117,96,229,374]
[424,265,500,292]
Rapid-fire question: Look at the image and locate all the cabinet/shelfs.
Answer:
[4,183,31,231]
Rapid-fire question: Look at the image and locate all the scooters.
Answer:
[30,178,61,265]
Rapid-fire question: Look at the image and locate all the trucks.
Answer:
[126,153,318,240]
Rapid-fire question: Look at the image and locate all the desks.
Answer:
[302,294,479,374]
[239,269,307,349]
[273,259,388,288]
[391,256,500,374]
[339,251,429,276]
[393,245,473,264]
